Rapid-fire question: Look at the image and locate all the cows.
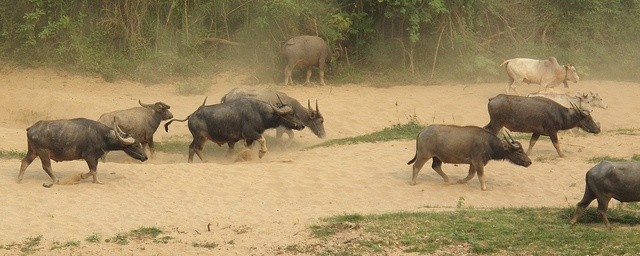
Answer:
[586,91,608,109]
[164,98,306,164]
[545,92,589,112]
[280,35,342,86]
[220,88,327,143]
[106,98,173,161]
[407,123,532,190]
[566,163,639,229]
[482,96,601,158]
[17,117,148,184]
[499,56,581,95]
